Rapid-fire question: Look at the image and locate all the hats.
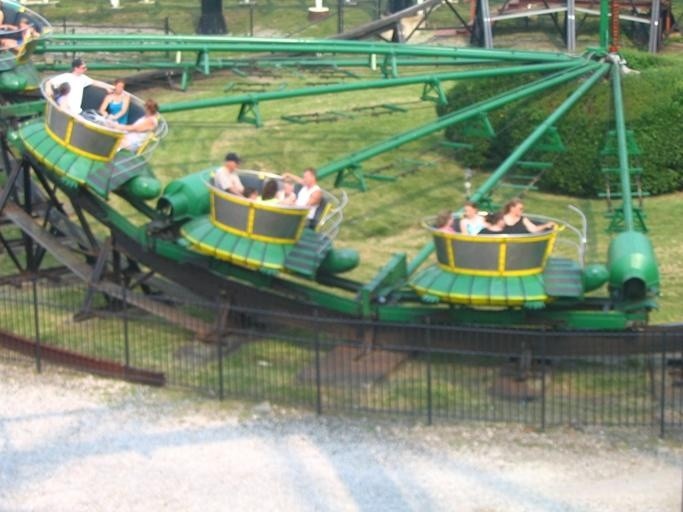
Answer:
[225,154,240,161]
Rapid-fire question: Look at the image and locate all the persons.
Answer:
[214,153,323,227]
[45,59,159,154]
[434,198,555,233]
[0,10,40,51]
